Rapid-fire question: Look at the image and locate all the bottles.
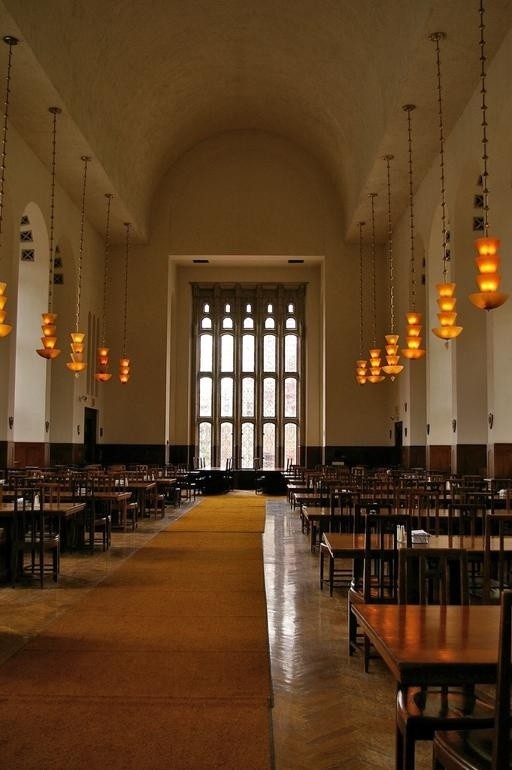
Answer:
[17,494,41,510]
[396,524,406,543]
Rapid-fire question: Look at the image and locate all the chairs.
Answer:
[280,456,512,598]
[347,514,413,674]
[1,457,233,589]
[431,589,512,770]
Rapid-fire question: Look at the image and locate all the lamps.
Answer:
[349,152,404,392]
[67,155,91,379]
[399,100,429,363]
[0,36,21,338]
[94,192,113,383]
[36,107,62,360]
[117,220,130,385]
[431,30,463,352]
[465,0,510,310]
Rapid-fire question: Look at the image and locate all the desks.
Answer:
[351,603,503,770]
